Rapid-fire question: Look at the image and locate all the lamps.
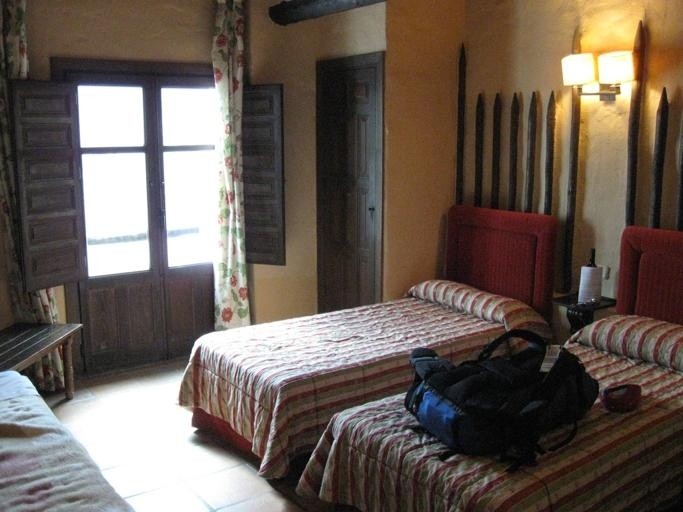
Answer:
[556,48,634,104]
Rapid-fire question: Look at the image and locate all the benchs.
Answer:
[0,319,83,402]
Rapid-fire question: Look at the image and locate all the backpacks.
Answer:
[403,327,599,473]
